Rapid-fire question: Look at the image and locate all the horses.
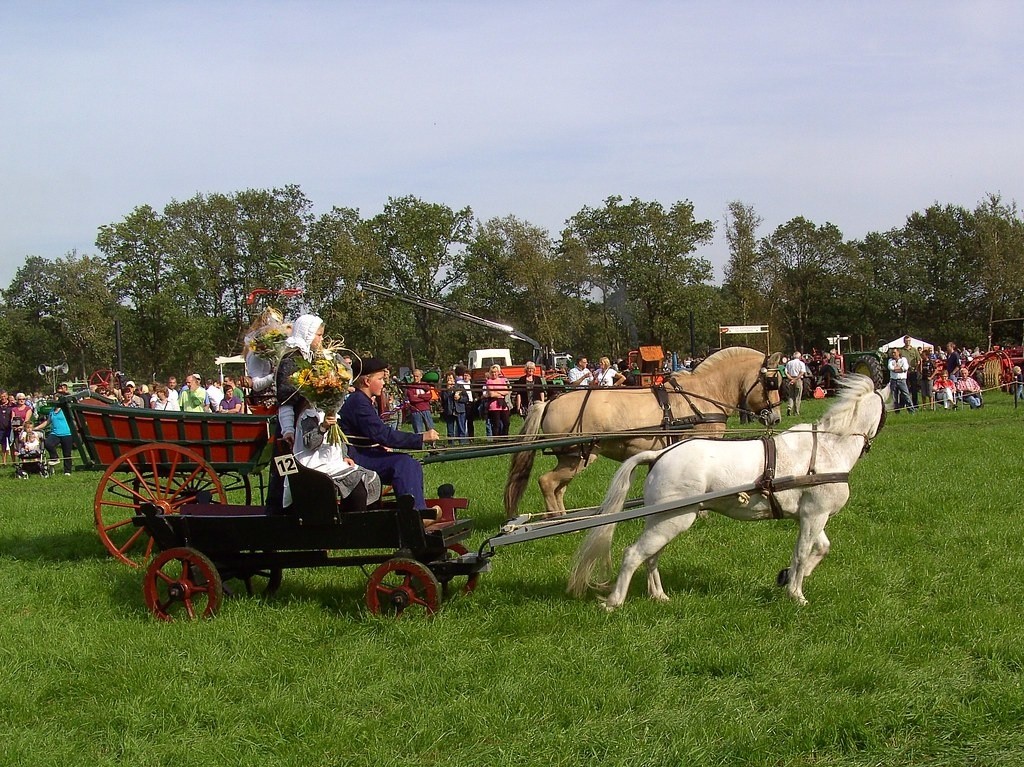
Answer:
[565,369,893,614]
[503,343,784,536]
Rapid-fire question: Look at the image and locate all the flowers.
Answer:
[289,349,354,444]
[242,318,294,370]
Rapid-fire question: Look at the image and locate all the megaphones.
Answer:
[38,364,53,376]
[54,362,69,374]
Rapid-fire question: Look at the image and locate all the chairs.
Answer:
[931,390,956,411]
[960,389,985,411]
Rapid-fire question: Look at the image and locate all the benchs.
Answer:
[279,440,345,525]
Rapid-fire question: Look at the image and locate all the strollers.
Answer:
[13,428,55,480]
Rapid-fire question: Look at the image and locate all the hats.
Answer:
[193,373,201,380]
[352,357,388,376]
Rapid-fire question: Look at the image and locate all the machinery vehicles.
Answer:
[356,281,574,418]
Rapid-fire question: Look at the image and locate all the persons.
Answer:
[0,373,244,478]
[244,314,443,526]
[568,351,806,416]
[889,336,1024,413]
[407,360,545,447]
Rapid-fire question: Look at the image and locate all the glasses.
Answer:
[18,398,25,400]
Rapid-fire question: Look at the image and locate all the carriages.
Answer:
[57,346,785,572]
[133,374,892,622]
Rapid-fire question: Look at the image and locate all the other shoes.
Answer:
[48,459,60,465]
[65,473,70,476]
